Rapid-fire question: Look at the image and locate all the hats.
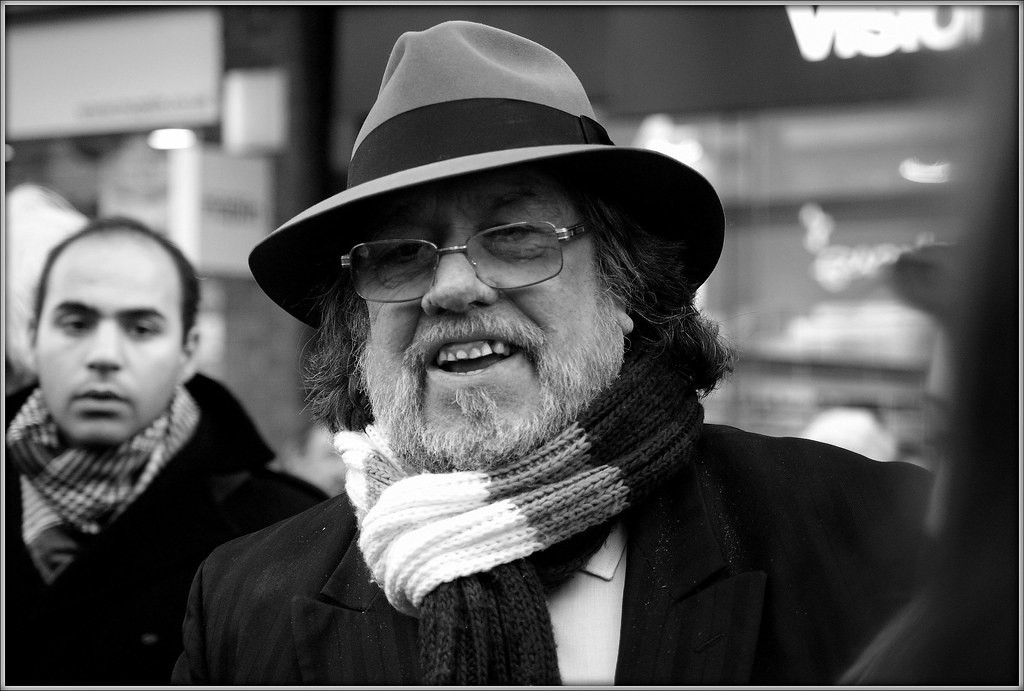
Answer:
[248,19,725,330]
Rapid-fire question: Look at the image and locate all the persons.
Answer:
[2,216,334,688]
[163,19,929,686]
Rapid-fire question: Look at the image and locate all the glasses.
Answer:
[340,219,590,303]
